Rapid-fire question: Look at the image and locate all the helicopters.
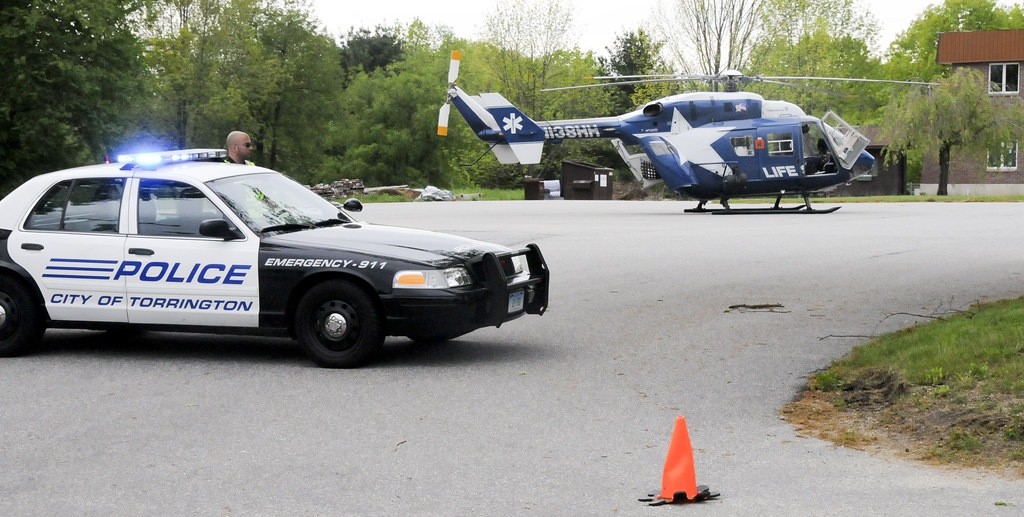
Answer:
[436,48,942,216]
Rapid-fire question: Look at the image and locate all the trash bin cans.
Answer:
[523,180,544,200]
[561,158,614,201]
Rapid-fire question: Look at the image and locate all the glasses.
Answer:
[234,143,252,149]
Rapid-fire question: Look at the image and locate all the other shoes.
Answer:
[815,171,825,175]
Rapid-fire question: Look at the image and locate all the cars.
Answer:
[0,149,551,358]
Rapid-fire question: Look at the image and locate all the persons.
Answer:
[213,131,266,228]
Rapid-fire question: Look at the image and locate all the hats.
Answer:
[803,124,810,129]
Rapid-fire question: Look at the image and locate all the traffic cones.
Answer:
[639,418,720,506]
